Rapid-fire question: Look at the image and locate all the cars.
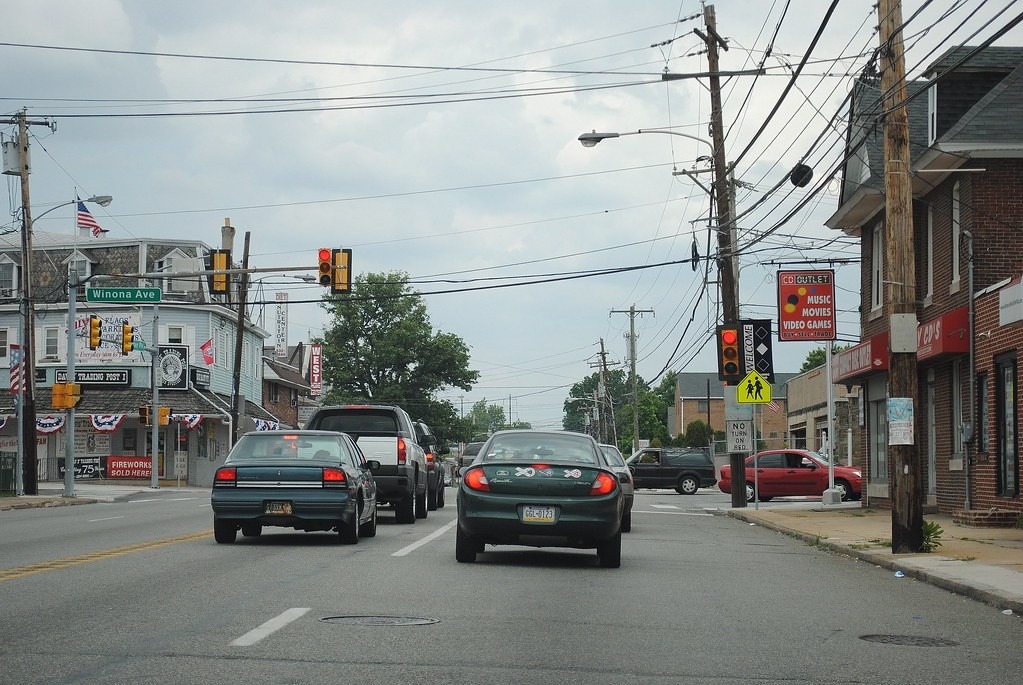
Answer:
[211,431,380,546]
[455,429,634,569]
[718,449,862,503]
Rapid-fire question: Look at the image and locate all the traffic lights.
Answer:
[122,322,135,356]
[159,406,173,426]
[716,324,746,380]
[89,315,102,350]
[318,248,332,286]
[64,384,84,407]
[138,405,149,424]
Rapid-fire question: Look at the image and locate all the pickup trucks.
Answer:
[625,447,717,495]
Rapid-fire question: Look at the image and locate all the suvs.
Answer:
[297,405,458,524]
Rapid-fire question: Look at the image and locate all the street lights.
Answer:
[232,273,317,451]
[576,130,747,509]
[21,195,113,495]
[564,397,613,445]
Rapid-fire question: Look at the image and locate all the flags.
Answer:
[201,339,214,365]
[76,197,101,237]
[766,400,781,414]
